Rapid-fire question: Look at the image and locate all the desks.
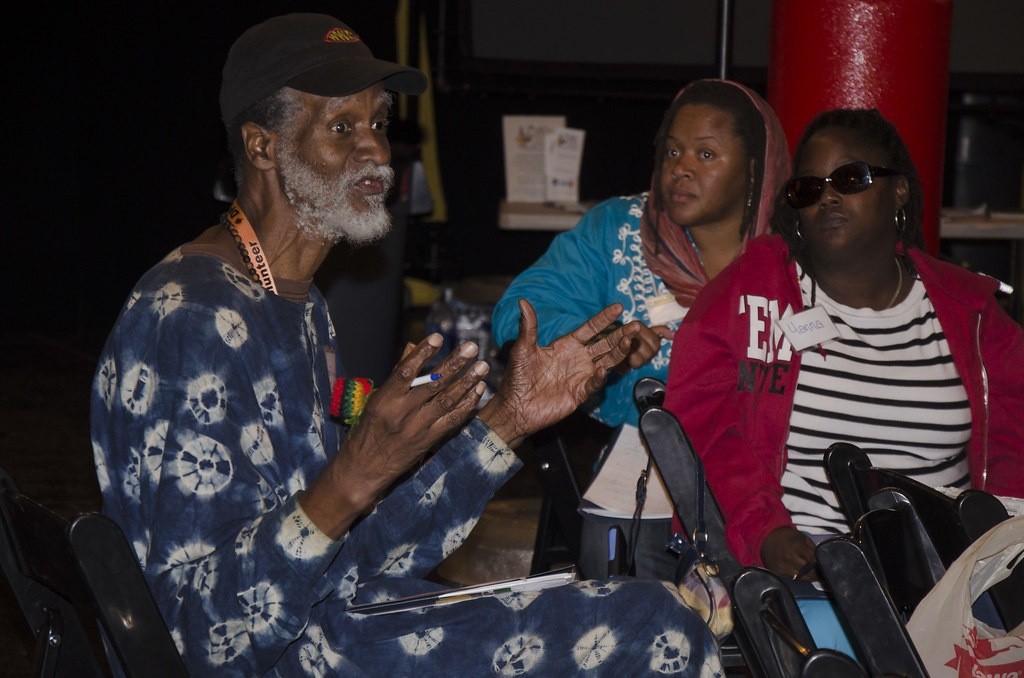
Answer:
[497,199,1024,327]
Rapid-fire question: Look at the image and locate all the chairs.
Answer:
[494,338,616,575]
[0,466,189,678]
[633,377,1024,678]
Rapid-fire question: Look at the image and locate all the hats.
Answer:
[220,13,429,124]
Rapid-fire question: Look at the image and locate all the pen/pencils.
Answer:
[411,373,444,387]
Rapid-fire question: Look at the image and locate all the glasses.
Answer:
[784,161,900,209]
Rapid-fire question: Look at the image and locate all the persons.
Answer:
[662,107,1024,582]
[90,13,724,678]
[491,78,795,580]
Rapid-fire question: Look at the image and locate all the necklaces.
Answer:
[882,258,903,309]
[218,200,279,297]
